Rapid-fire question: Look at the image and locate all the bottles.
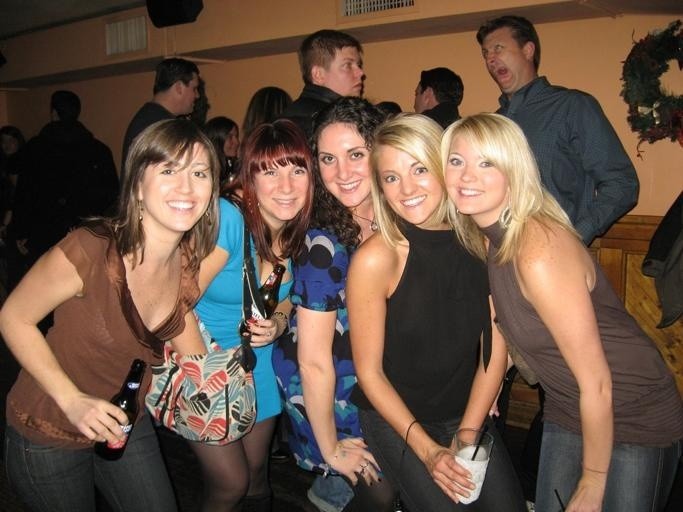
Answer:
[92,357,147,460]
[244,263,286,335]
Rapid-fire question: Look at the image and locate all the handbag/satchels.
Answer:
[144,342,257,445]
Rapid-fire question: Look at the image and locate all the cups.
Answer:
[452,427,494,505]
